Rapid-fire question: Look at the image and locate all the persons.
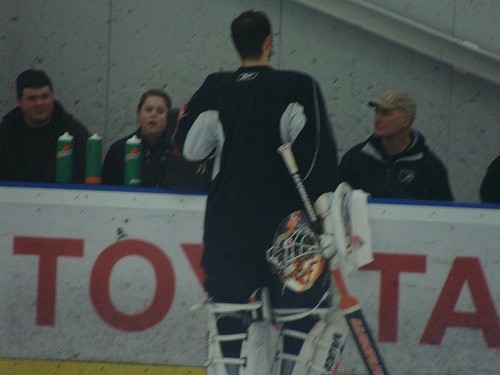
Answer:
[170,8,345,375]
[480,154,500,204]
[104,87,197,190]
[0,69,93,184]
[336,92,453,203]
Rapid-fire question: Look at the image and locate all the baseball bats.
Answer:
[277,141,386,375]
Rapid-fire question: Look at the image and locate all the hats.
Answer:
[368,91,418,121]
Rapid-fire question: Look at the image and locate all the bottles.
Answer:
[125,134,142,185]
[85,133,103,184]
[56,132,73,183]
